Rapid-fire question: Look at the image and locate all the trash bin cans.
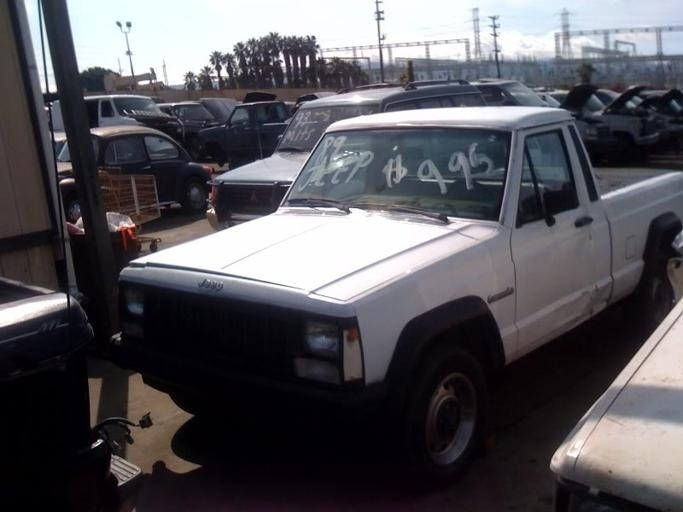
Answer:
[51,225,138,338]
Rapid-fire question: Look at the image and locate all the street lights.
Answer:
[116,21,139,95]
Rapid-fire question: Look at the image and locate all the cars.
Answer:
[549,296,682,510]
[469,78,683,165]
[205,80,488,233]
[55,127,213,227]
[46,91,341,169]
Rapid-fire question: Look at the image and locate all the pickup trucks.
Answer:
[0,277,142,509]
[109,104,682,490]
